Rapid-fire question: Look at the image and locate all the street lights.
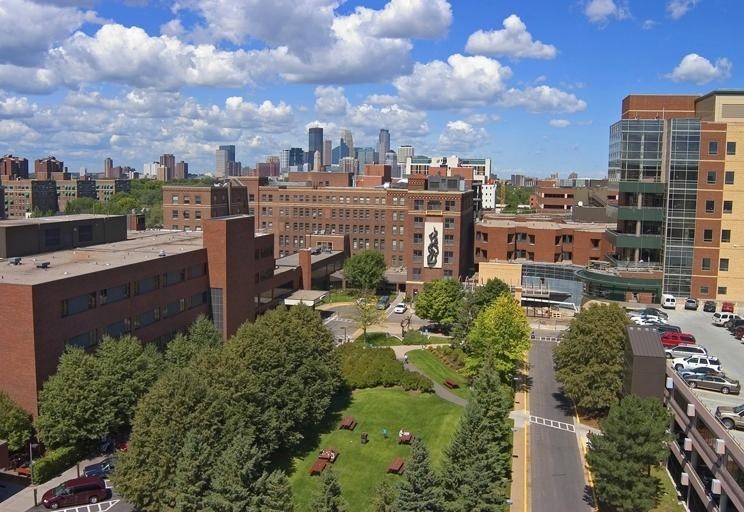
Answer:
[26,442,37,487]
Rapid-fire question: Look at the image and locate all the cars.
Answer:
[421,322,444,332]
[722,302,735,313]
[625,308,744,395]
[703,300,718,313]
[41,438,132,509]
[393,302,407,315]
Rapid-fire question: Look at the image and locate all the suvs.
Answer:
[685,298,700,310]
[715,404,744,431]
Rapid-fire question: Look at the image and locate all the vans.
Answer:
[375,295,390,310]
[661,293,677,309]
